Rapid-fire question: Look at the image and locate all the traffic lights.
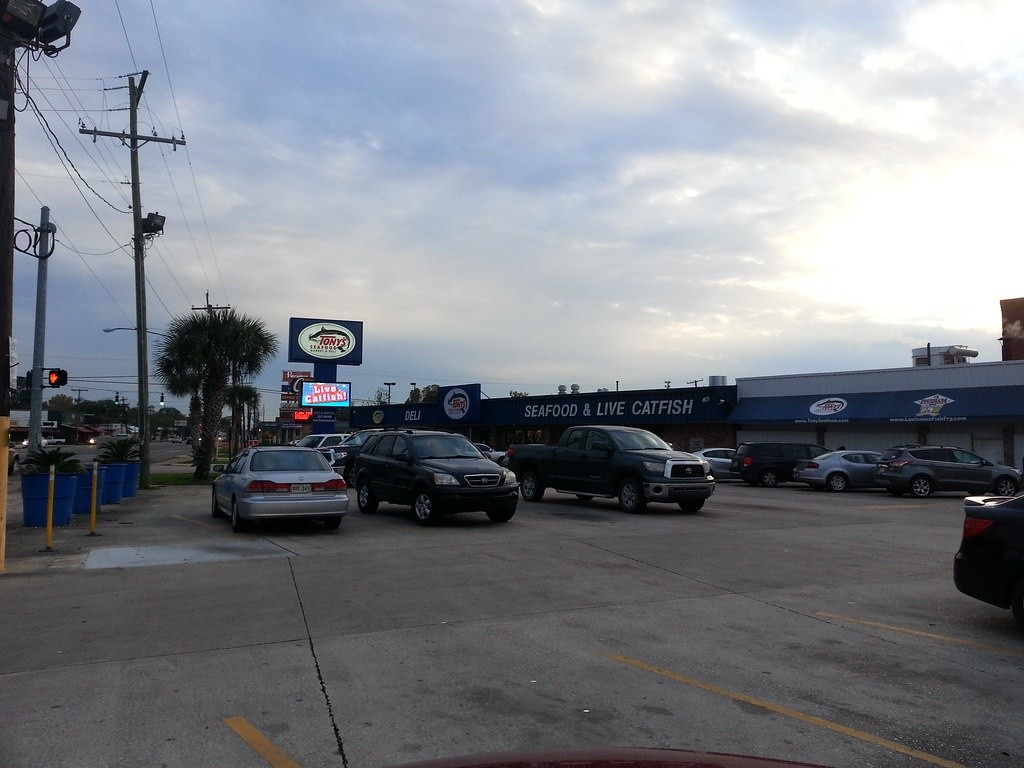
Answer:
[115,395,119,406]
[48,370,68,386]
[159,396,164,405]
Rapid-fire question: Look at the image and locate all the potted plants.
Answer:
[21,446,80,528]
[98,438,141,505]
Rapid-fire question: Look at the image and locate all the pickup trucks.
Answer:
[508,425,716,514]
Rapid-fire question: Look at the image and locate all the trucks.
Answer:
[46,436,67,445]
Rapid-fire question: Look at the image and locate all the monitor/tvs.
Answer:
[301,381,351,409]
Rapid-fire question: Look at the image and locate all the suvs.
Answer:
[729,441,834,488]
[354,428,520,527]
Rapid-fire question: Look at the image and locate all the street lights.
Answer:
[384,382,396,404]
[410,382,416,389]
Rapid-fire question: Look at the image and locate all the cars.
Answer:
[212,446,349,534]
[294,428,386,489]
[953,493,1024,638]
[173,437,182,443]
[873,444,1024,499]
[792,450,883,493]
[691,447,742,479]
[22,436,48,449]
[465,443,507,468]
[8,445,20,475]
